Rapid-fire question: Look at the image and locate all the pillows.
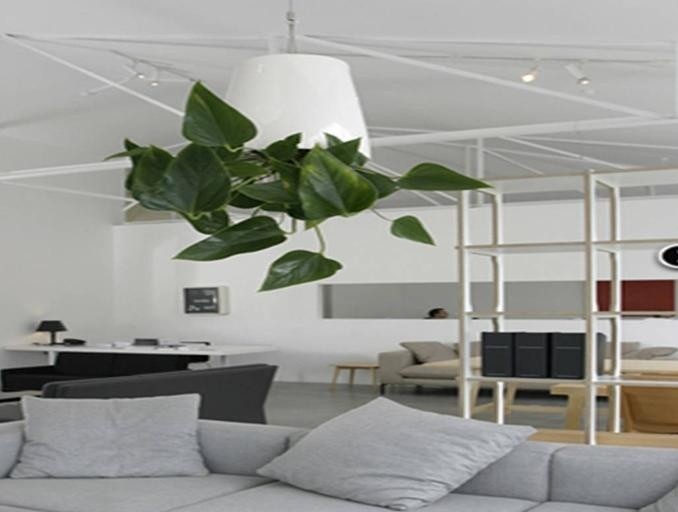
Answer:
[5,391,212,481]
[398,338,459,369]
[605,340,637,359]
[625,345,677,360]
[254,394,541,508]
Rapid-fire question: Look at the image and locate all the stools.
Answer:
[550,381,614,433]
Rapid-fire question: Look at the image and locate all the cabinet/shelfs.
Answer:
[453,170,678,450]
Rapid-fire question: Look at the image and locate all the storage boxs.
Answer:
[480,322,608,388]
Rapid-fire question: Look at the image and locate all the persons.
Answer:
[423,308,448,320]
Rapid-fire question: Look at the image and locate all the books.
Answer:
[134,337,159,346]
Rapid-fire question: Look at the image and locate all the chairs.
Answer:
[613,372,677,435]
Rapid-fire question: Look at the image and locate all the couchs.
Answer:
[372,334,678,399]
[0,416,676,510]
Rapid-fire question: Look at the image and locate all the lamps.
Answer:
[31,317,69,352]
[514,54,592,91]
[117,56,164,90]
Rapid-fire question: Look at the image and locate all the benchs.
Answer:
[41,364,276,426]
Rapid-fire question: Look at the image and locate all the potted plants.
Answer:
[96,51,492,293]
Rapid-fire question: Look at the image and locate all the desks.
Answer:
[2,325,287,369]
[329,361,387,391]
